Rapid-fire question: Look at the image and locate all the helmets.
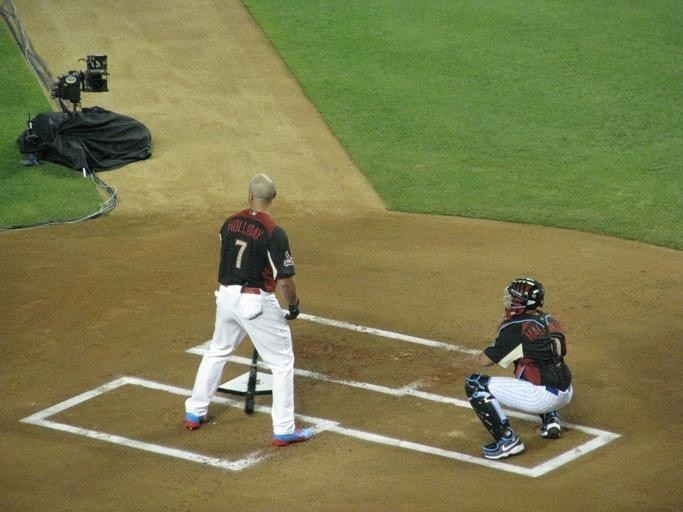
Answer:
[502,277,545,321]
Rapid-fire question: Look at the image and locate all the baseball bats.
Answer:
[245,348,258,413]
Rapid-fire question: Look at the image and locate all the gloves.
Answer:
[284,299,299,319]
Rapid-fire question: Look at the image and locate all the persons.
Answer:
[183,172,314,447]
[465,276,573,460]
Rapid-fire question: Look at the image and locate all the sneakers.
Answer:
[183,412,208,430]
[481,433,525,459]
[538,414,561,439]
[271,429,312,446]
